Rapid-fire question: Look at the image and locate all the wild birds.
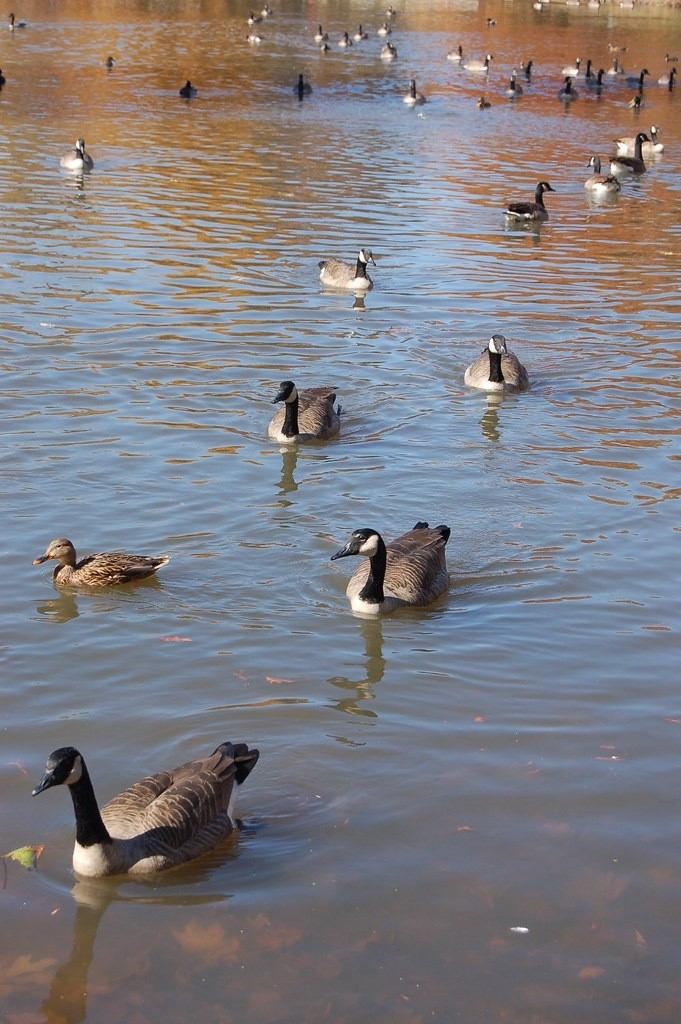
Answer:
[292,73,313,95]
[330,520,453,616]
[243,4,274,27]
[484,17,498,25]
[30,740,260,879]
[59,137,96,168]
[99,55,117,69]
[317,247,377,290]
[7,11,28,27]
[267,379,344,444]
[463,334,530,393]
[31,537,171,588]
[312,3,399,57]
[243,33,266,43]
[500,180,557,222]
[402,77,427,106]
[445,39,680,111]
[583,125,665,191]
[178,79,200,99]
